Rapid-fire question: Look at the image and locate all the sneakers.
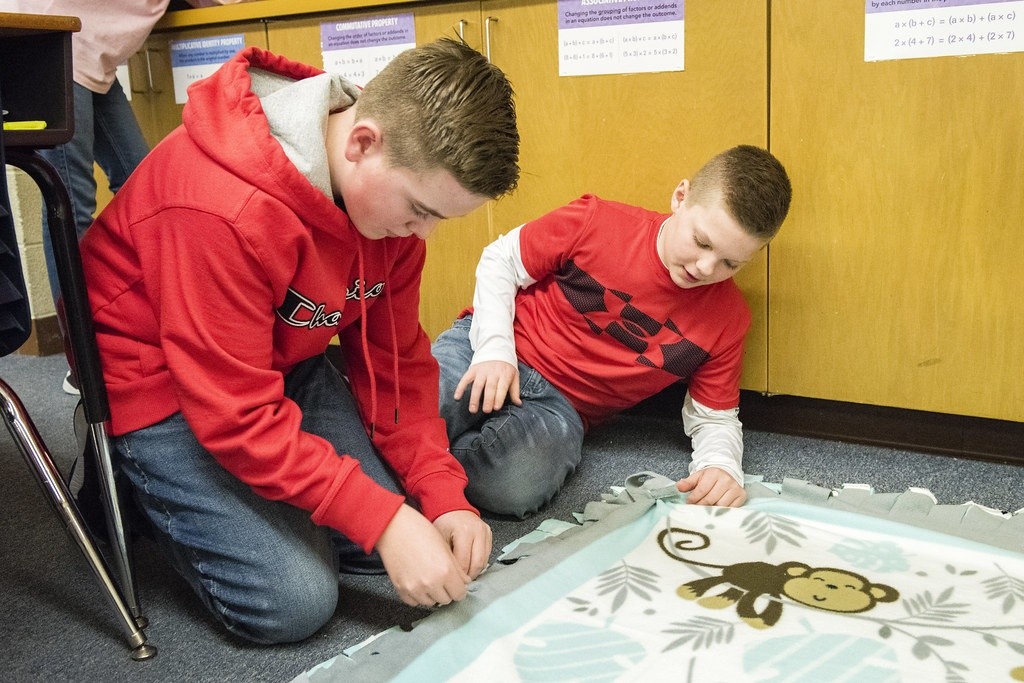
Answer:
[68,401,111,544]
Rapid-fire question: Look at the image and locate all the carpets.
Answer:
[285,462,1022,683]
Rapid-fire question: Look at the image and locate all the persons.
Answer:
[430,146,793,523]
[0,0,247,397]
[59,30,519,649]
